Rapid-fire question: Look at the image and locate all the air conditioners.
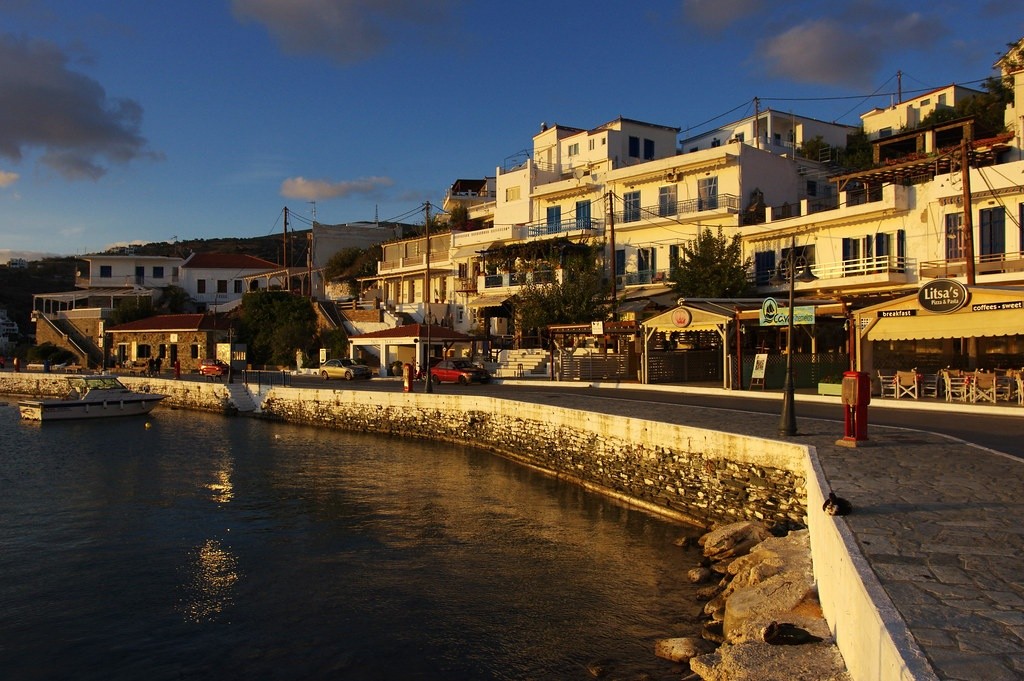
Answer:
[667,172,678,181]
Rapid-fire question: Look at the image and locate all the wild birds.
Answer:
[761,621,812,645]
[823,492,849,517]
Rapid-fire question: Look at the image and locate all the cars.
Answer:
[198,359,230,376]
[319,358,372,381]
[431,360,491,386]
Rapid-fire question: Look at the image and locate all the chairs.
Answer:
[877,367,1024,406]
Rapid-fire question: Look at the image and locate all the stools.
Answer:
[495,367,501,377]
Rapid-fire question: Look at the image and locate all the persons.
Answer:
[147,355,162,377]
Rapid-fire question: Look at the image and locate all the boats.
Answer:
[17,374,170,423]
